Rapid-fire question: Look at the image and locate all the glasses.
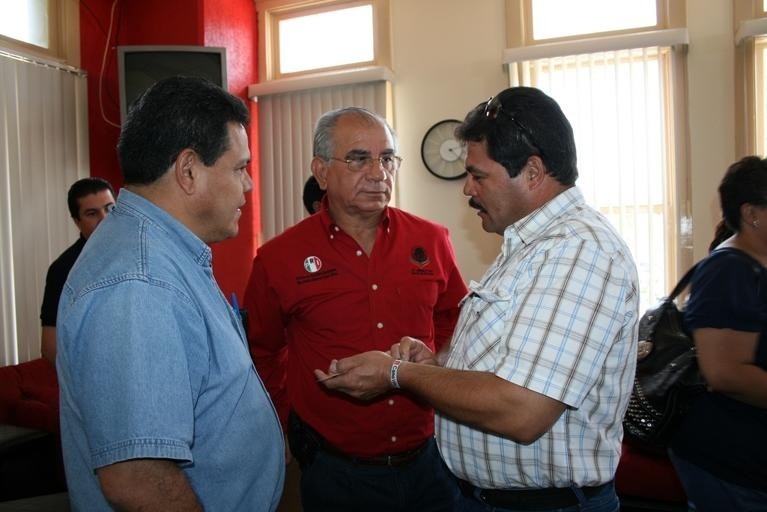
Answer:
[484,94,536,137]
[327,153,405,174]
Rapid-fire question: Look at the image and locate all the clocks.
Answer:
[421,118,468,180]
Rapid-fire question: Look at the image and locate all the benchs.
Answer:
[0,356,684,512]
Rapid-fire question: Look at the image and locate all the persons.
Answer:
[707,221,733,251]
[54,75,287,512]
[241,107,472,510]
[661,155,767,511]
[39,176,115,366]
[303,174,328,217]
[312,86,639,512]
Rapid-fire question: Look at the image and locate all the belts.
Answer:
[298,420,438,465]
[451,474,601,510]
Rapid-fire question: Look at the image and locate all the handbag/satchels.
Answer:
[621,246,766,447]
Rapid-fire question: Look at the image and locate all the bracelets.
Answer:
[390,358,402,390]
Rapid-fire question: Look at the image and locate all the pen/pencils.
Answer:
[230,292,242,321]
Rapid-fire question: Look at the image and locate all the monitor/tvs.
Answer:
[117,44,227,132]
